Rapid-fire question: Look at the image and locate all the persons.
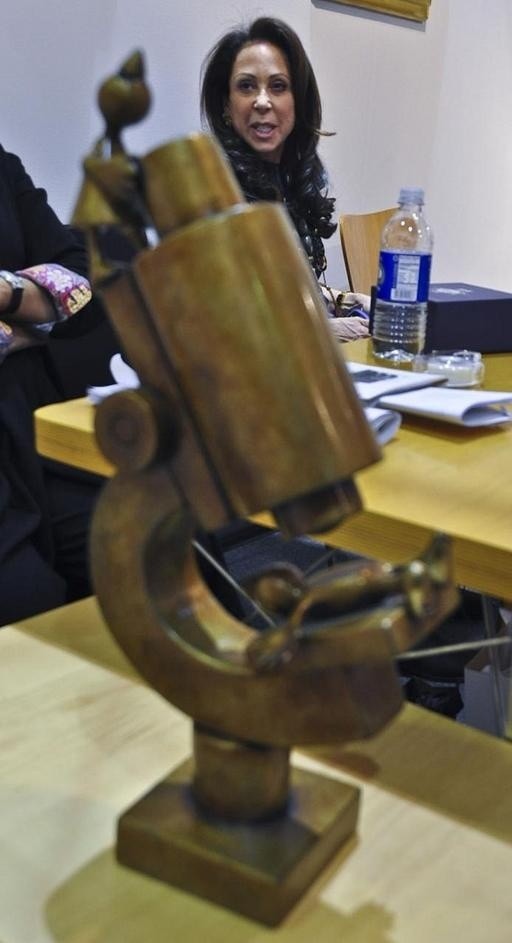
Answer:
[0,142,123,628]
[198,16,341,280]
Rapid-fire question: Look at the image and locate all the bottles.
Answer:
[370,188,434,363]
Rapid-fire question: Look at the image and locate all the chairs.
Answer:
[339,208,418,299]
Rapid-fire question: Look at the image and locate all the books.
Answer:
[87,355,402,449]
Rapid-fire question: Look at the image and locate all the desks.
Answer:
[35,336,510,743]
[0,595,509,942]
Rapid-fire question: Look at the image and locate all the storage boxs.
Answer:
[368,282,511,354]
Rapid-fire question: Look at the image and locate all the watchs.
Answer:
[0,270,26,314]
